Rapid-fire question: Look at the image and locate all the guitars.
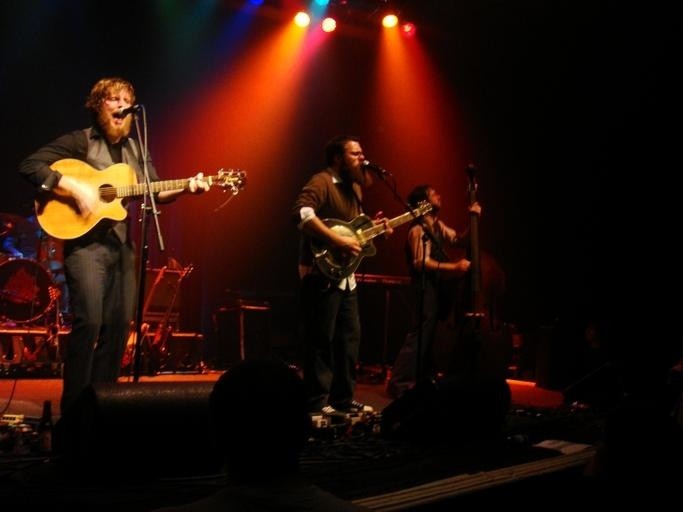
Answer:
[33,157,246,239]
[306,199,434,278]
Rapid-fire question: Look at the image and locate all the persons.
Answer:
[1,210,69,334]
[12,75,211,426]
[291,137,374,425]
[34,381,167,512]
[167,356,383,512]
[403,181,482,375]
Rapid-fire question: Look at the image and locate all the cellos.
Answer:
[430,165,493,376]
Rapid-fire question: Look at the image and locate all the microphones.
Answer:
[118,104,139,117]
[362,160,392,175]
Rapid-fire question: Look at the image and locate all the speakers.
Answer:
[52,382,227,482]
[378,371,510,442]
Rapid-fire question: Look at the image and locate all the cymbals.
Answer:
[0,212,32,236]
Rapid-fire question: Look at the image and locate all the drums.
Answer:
[0,255,55,324]
[39,239,63,271]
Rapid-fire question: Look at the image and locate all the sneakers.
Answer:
[315,400,374,424]
